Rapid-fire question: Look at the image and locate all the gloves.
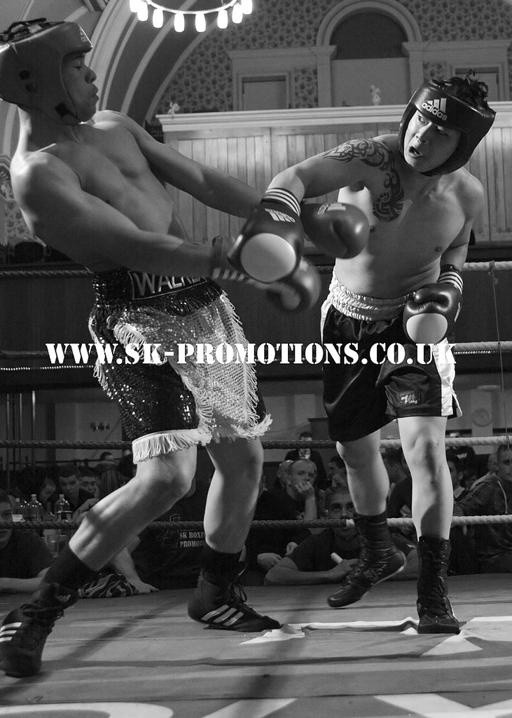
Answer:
[212,189,370,313]
[403,263,464,343]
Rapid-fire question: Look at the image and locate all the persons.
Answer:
[0,430,512,594]
[228,76,497,635]
[1,16,371,677]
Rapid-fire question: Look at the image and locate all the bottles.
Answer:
[11,497,28,523]
[26,494,44,522]
[53,493,71,523]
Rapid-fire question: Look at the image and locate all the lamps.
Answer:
[127,0,254,36]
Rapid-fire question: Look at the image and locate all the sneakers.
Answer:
[328,538,461,632]
[188,567,280,631]
[0,583,77,677]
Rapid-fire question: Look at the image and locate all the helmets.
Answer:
[399,77,495,176]
[0,18,93,125]
